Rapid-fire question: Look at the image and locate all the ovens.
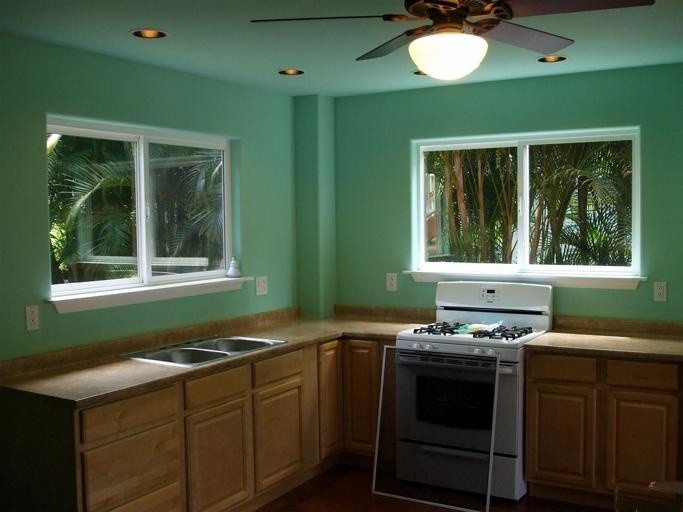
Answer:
[395,351,521,499]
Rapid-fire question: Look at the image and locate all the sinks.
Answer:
[120,348,229,368]
[189,334,288,352]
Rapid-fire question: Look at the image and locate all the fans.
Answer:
[251,0,657,61]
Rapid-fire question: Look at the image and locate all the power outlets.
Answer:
[386,273,397,293]
[27,306,39,330]
[256,276,268,295]
[654,282,667,301]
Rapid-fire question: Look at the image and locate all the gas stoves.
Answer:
[397,321,532,344]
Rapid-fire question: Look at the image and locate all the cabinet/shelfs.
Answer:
[523,349,683,512]
[2,386,185,512]
[185,350,305,512]
[318,336,382,463]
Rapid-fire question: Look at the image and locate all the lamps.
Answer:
[407,29,489,81]
